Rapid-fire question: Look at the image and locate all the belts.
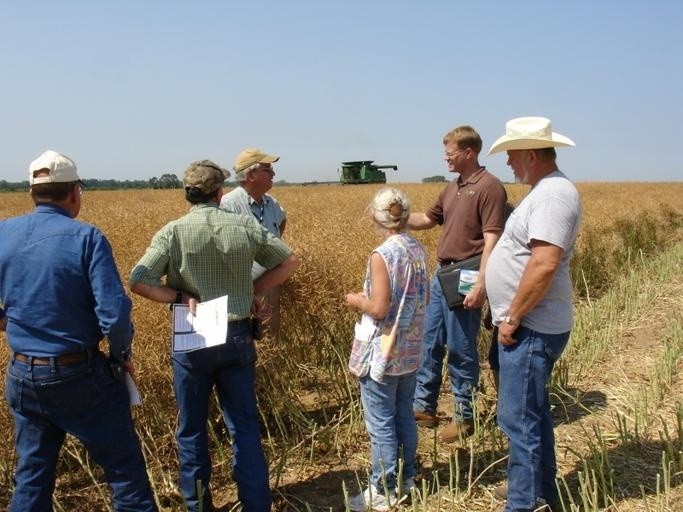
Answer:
[227,321,250,330]
[14,354,87,367]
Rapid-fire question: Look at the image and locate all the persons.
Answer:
[343,186,431,512]
[217,147,287,338]
[128,158,299,512]
[484,115,582,511]
[405,125,509,444]
[0,147,161,511]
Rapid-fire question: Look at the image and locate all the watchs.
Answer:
[505,312,521,327]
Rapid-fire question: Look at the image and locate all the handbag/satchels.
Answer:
[436,254,487,311]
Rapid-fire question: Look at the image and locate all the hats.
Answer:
[485,116,576,158]
[233,147,280,174]
[28,150,80,186]
[183,159,231,195]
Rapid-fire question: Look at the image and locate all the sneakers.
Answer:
[396,478,416,496]
[440,421,475,443]
[343,484,396,511]
[412,410,436,428]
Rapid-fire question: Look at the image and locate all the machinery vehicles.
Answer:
[337,159,399,185]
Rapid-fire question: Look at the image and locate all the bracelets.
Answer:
[173,289,183,305]
